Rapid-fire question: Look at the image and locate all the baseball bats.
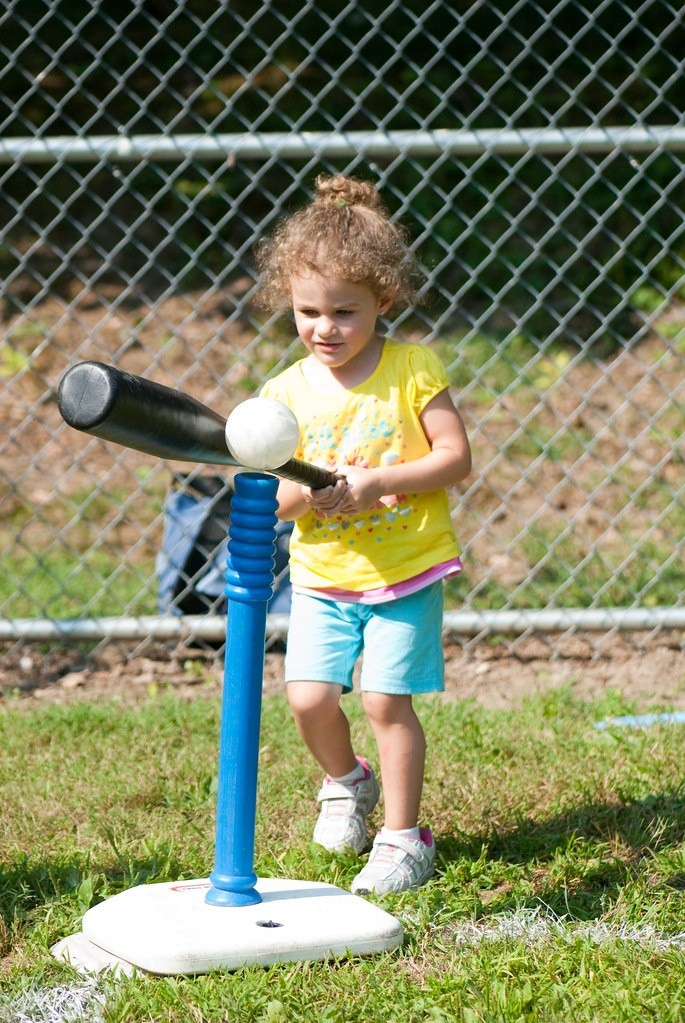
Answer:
[56,356,340,490]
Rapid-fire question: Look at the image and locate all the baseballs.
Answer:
[222,394,302,474]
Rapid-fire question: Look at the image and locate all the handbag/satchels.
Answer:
[157,473,294,652]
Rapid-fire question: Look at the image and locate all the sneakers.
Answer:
[313,753,380,856]
[352,828,434,897]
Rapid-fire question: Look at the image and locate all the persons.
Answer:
[257,177,472,894]
